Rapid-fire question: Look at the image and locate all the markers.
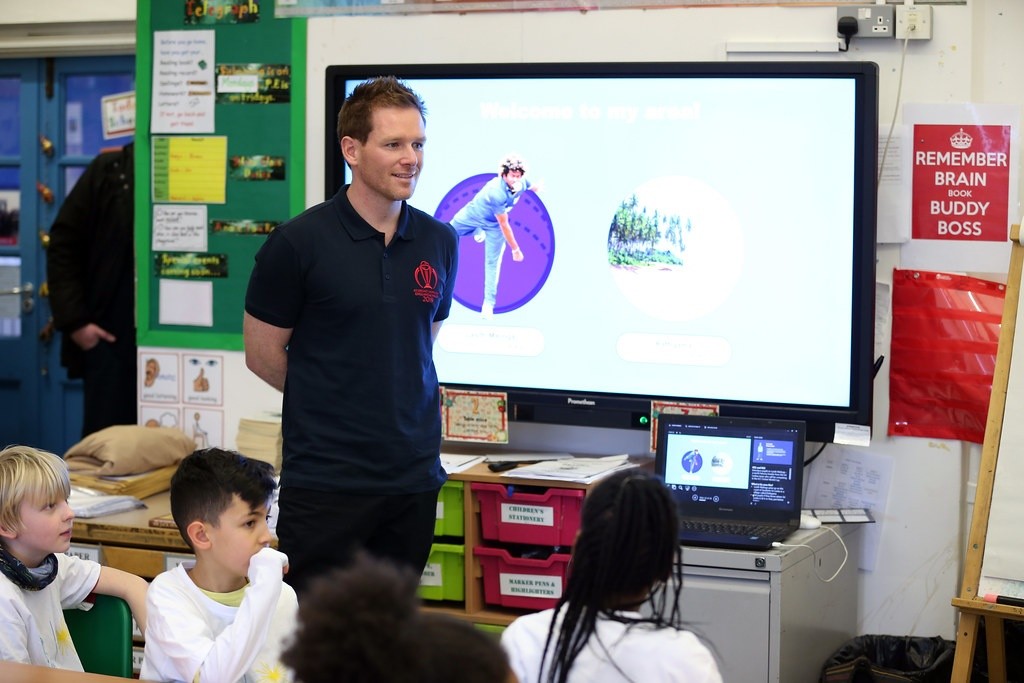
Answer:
[984,593,1024,607]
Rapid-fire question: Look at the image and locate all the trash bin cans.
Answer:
[818,631,957,683]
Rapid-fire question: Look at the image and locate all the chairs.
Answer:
[63,593,133,678]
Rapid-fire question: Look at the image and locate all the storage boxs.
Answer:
[471,482,585,545]
[433,480,465,536]
[413,544,465,602]
[473,545,574,609]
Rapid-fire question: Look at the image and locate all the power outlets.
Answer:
[835,5,897,39]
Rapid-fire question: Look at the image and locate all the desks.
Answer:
[62,481,196,584]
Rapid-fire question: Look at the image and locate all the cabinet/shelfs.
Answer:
[406,451,658,636]
[638,517,861,683]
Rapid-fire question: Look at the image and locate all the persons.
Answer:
[243,76,460,604]
[47,142,137,440]
[449,158,537,315]
[0,443,150,683]
[498,470,722,682]
[280,553,518,682]
[139,448,300,683]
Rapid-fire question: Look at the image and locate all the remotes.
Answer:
[487,462,518,472]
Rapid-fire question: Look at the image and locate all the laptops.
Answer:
[651,414,806,551]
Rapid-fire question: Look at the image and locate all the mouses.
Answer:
[800,515,821,529]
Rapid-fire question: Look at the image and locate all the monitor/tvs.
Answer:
[326,60,878,443]
